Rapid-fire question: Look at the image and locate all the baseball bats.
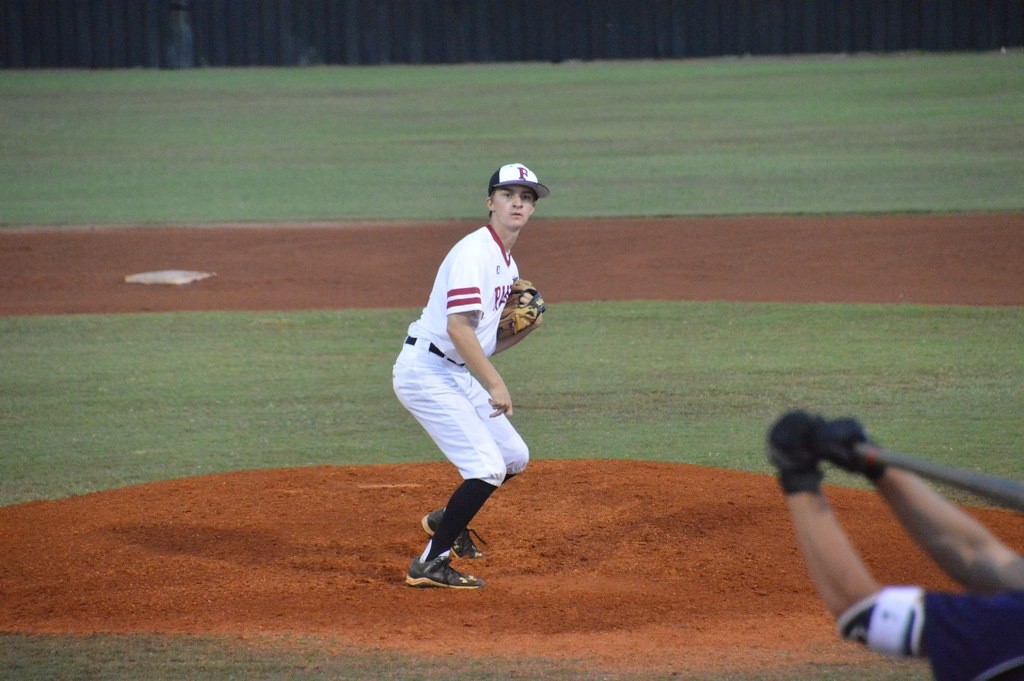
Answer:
[848,437,1024,510]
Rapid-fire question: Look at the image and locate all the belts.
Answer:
[405,336,465,367]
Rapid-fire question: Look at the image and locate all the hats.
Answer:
[489,163,550,198]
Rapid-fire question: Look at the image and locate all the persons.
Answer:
[392,163,551,589]
[766,410,1024,681]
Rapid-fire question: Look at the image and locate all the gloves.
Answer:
[765,409,825,495]
[819,415,886,483]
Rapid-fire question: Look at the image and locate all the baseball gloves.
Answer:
[497,279,546,342]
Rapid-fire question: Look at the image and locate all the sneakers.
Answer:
[405,553,483,589]
[422,505,488,559]
